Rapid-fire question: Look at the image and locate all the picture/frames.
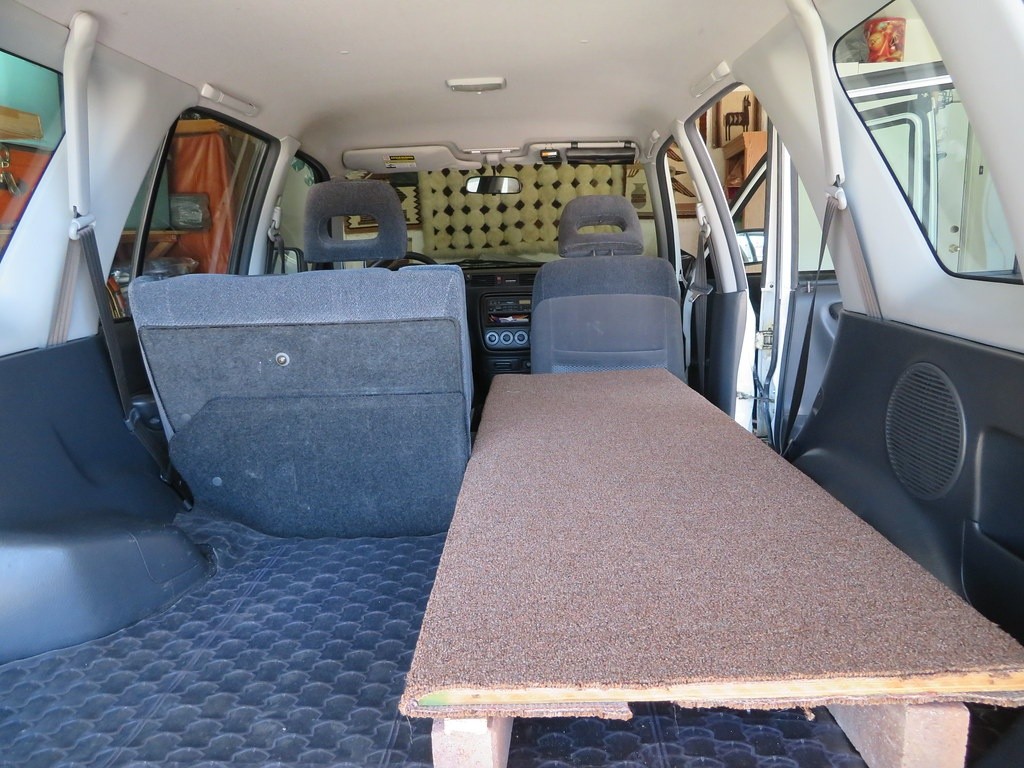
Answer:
[622,111,707,219]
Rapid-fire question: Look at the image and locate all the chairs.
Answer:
[130,179,474,538]
[529,206,685,383]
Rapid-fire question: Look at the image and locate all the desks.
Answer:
[396,366,1024,768]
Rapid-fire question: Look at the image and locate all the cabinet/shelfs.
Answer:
[722,129,767,230]
